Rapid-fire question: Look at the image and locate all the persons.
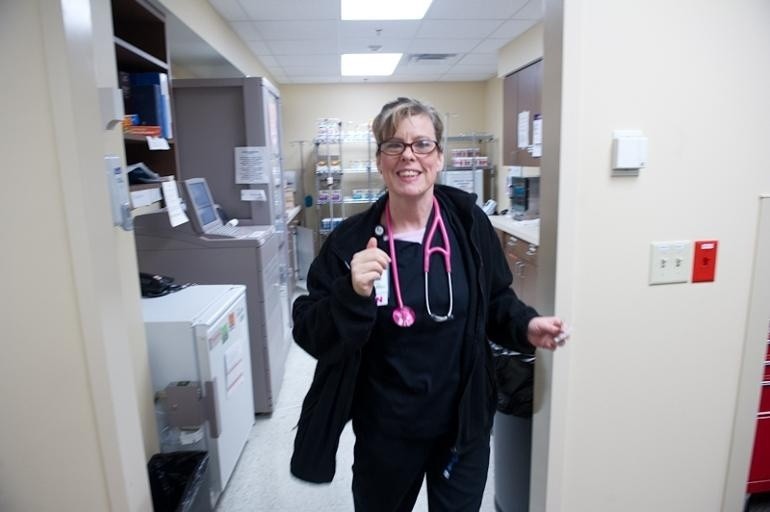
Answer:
[290,95,570,512]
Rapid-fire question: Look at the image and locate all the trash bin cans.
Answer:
[147,449,213,512]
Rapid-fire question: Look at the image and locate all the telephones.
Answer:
[139,272,174,296]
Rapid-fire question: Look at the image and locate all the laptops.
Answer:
[179,177,252,238]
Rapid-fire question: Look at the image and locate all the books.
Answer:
[115,68,178,184]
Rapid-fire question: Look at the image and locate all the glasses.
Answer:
[378,137,442,156]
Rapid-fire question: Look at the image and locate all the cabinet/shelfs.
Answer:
[492,225,539,308]
[314,138,494,246]
[111,0,183,218]
